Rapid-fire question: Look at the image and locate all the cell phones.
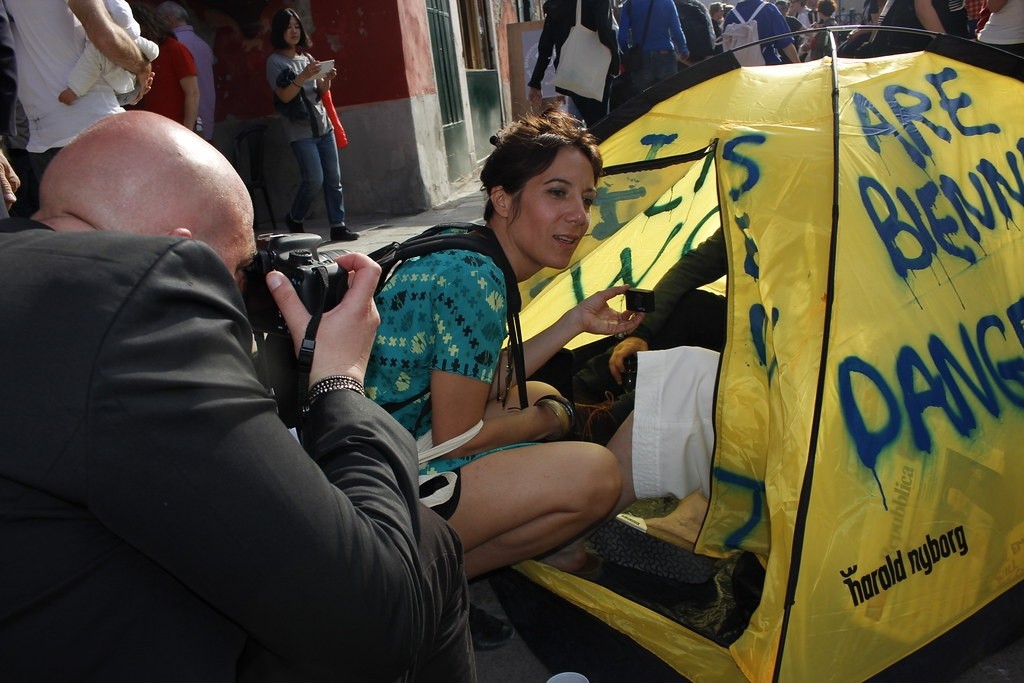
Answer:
[316,60,335,74]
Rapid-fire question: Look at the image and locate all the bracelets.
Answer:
[537,399,569,438]
[307,375,365,398]
[534,395,574,425]
[292,80,301,87]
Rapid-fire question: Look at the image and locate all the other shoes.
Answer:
[286,213,306,232]
[470,603,513,649]
[330,225,359,241]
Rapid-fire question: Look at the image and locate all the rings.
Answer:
[615,332,627,339]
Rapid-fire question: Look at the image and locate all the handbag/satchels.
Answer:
[556,0,613,101]
[628,45,642,71]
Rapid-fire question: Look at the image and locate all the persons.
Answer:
[4,0,155,153]
[159,1,216,144]
[266,8,360,241]
[528,0,1024,145]
[0,1,22,221]
[942,476,1003,533]
[0,109,478,683]
[541,225,729,572]
[130,3,200,132]
[364,112,644,651]
[58,0,160,106]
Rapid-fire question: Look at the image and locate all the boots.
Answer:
[618,490,708,551]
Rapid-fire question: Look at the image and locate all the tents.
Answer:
[504,24,1024,683]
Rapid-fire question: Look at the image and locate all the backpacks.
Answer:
[251,222,528,429]
[713,1,769,66]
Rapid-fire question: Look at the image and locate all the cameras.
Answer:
[243,231,356,340]
